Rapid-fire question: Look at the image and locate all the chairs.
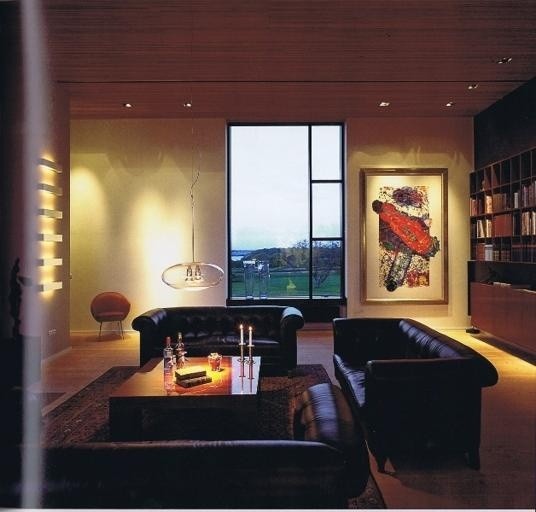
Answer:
[90,292,130,341]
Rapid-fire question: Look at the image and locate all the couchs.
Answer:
[42,384,369,509]
[131,307,305,376]
[332,318,499,472]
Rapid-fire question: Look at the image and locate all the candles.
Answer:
[240,324,244,345]
[249,326,255,346]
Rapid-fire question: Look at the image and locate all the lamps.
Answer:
[161,262,224,291]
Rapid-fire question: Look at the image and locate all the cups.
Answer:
[243,260,269,300]
[208,355,222,372]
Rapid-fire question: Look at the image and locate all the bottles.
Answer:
[163,332,183,374]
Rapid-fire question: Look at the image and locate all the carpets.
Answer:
[42,363,386,509]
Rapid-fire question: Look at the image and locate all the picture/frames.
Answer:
[359,168,449,305]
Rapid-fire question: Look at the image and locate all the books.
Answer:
[175,377,212,388]
[468,180,536,263]
[175,365,206,382]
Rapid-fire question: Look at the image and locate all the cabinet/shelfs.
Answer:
[467,147,535,356]
